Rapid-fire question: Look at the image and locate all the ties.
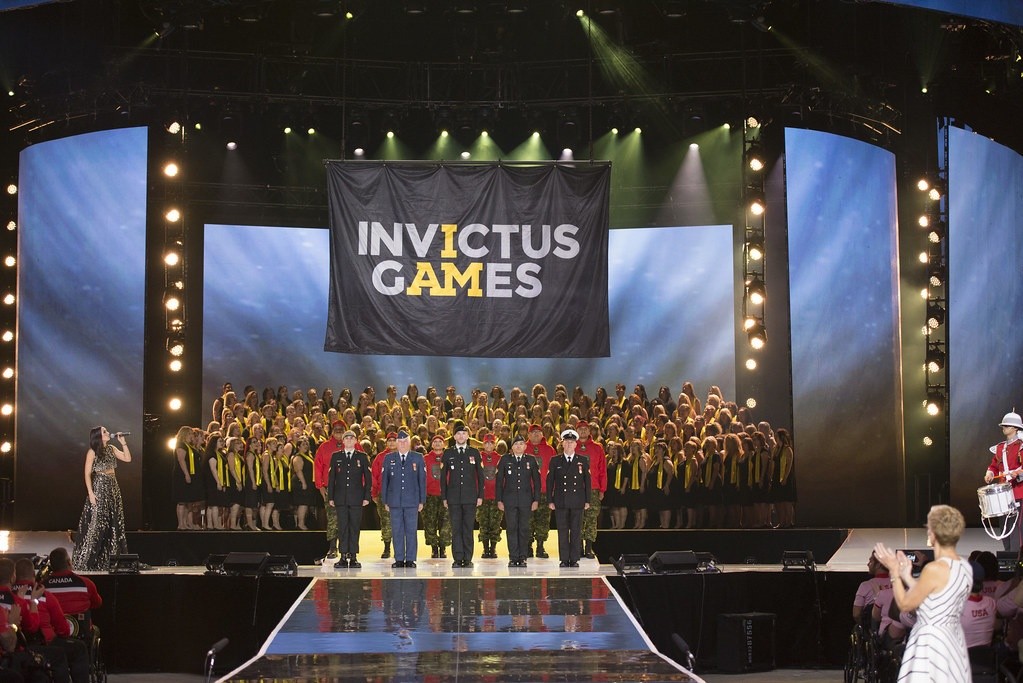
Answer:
[347,452,351,460]
[402,455,405,464]
[568,457,571,466]
[517,457,520,464]
[459,447,463,455]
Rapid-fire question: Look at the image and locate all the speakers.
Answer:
[647,550,698,573]
[896,548,934,573]
[718,611,776,672]
[224,551,272,575]
[0,553,37,569]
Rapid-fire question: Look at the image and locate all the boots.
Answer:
[326,539,594,559]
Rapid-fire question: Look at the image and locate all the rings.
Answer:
[900,562,903,565]
[878,554,882,559]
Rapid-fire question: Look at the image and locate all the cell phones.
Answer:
[903,551,917,563]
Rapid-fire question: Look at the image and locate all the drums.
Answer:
[977,483,1020,518]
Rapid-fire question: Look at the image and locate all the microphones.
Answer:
[110,432,131,438]
[672,632,694,661]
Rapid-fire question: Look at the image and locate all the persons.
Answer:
[0,547,103,683]
[496,434,541,567]
[221,580,696,683]
[960,550,1023,683]
[874,505,975,683]
[382,430,426,568]
[546,429,591,568]
[70,425,158,571]
[853,550,931,644]
[173,383,796,559]
[440,427,484,569]
[984,407,1023,551]
[328,430,371,568]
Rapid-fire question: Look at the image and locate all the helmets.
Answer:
[999,408,1023,430]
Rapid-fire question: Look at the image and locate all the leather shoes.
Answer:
[463,561,473,568]
[453,561,462,568]
[349,560,361,568]
[509,561,527,567]
[334,559,348,568]
[392,561,404,568]
[560,561,579,568]
[405,561,416,568]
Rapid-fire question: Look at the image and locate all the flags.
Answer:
[324,158,614,358]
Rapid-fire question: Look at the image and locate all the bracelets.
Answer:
[121,443,128,446]
[890,577,901,582]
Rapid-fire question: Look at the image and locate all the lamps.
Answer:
[780,550,814,571]
[161,110,186,356]
[743,100,769,351]
[618,551,717,573]
[108,553,139,574]
[917,171,947,415]
[205,552,300,577]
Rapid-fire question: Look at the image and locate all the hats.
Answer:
[332,420,346,427]
[386,432,397,440]
[528,424,542,432]
[397,431,408,439]
[453,425,467,435]
[511,434,526,446]
[560,429,580,441]
[576,420,590,429]
[483,434,495,441]
[342,430,356,440]
[432,435,445,442]
